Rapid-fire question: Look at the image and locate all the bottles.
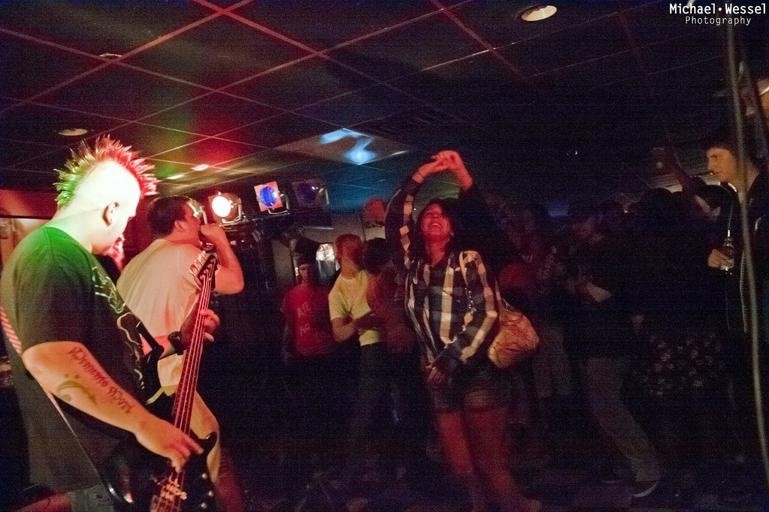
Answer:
[718,225,737,278]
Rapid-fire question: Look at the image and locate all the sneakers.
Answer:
[631,478,660,498]
[600,470,629,484]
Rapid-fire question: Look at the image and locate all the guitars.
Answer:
[103,245,218,512]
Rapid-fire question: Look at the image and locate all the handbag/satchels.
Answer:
[484,299,541,368]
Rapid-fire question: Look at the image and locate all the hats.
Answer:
[297,254,316,266]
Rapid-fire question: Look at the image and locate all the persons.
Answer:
[266,131,766,512]
[117,194,249,511]
[0,132,219,511]
